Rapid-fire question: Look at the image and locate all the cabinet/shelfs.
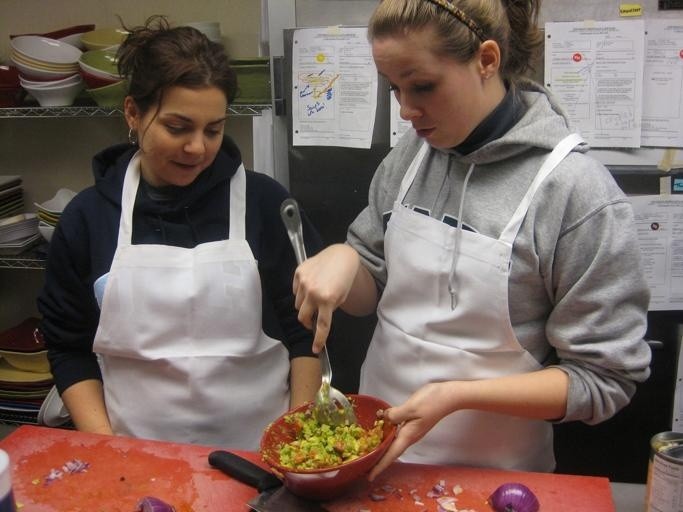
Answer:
[0,96,289,431]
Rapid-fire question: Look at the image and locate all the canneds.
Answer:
[645,430,682,511]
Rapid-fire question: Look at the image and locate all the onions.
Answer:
[138,497,174,510]
[486,482,541,512]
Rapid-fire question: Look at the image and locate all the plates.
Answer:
[0,172,80,255]
[0,365,73,428]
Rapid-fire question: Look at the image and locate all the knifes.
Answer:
[206,449,332,512]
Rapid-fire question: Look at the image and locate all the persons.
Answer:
[293,0,652,472]
[36,15,321,452]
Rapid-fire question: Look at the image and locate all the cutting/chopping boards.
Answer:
[0,423,617,510]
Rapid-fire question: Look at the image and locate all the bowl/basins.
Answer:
[0,316,52,373]
[0,22,220,108]
[258,396,398,498]
